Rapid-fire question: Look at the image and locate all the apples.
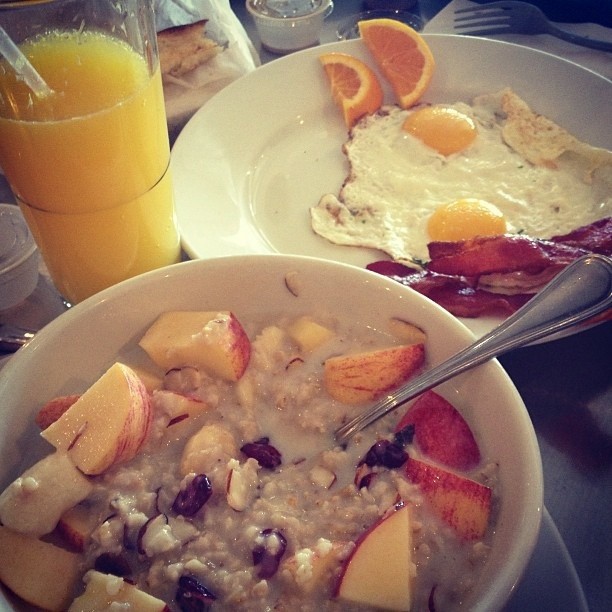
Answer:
[331,500,412,607]
[401,458,494,540]
[40,362,208,475]
[140,310,251,383]
[394,389,480,472]
[0,526,82,608]
[68,567,167,607]
[325,345,424,406]
[59,504,100,547]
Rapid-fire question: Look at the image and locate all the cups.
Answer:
[0,0,182,309]
[335,9,424,41]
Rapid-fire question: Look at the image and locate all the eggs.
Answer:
[308,88,612,266]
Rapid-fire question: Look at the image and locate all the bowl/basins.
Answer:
[0,252,545,611]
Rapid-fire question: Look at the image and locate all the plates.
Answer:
[170,31,612,348]
[502,508,590,611]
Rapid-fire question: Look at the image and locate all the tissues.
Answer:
[154,1,263,120]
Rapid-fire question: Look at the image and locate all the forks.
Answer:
[454,0,611,52]
